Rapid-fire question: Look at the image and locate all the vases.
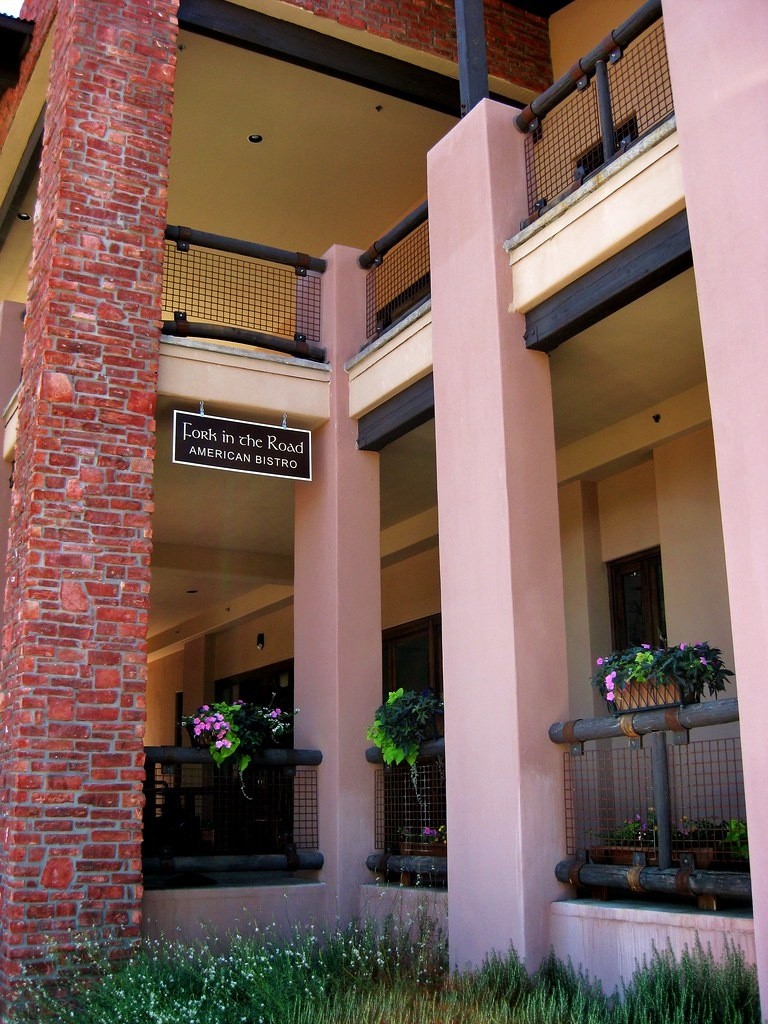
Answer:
[397,825,447,844]
[210,763,241,785]
[585,845,734,911]
[614,672,701,718]
[400,842,447,886]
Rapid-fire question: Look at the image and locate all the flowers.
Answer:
[178,695,300,772]
[584,806,749,860]
[591,641,735,704]
[366,692,444,767]
